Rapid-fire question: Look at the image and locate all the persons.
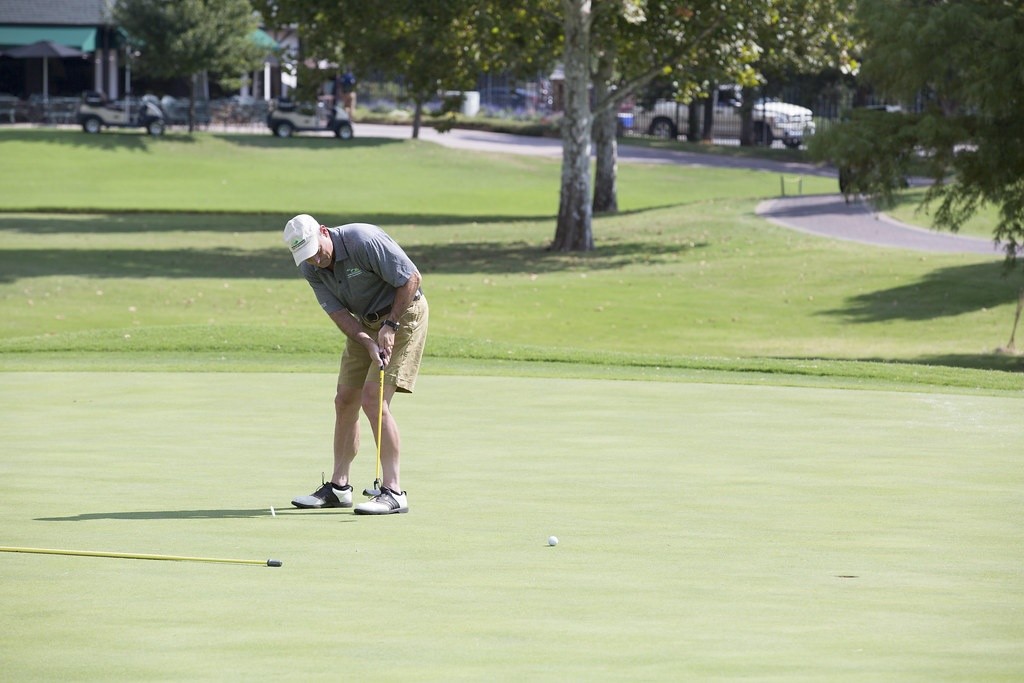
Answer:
[284,213,428,515]
[341,63,358,123]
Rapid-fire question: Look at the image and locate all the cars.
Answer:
[864,103,980,159]
[75,89,353,139]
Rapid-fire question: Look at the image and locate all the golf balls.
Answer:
[548,535,559,546]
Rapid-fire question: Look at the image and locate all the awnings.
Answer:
[112,26,280,50]
[0,25,96,51]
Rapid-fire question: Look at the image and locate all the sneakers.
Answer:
[353,486,410,516]
[291,471,354,509]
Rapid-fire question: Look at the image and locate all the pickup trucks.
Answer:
[633,76,817,151]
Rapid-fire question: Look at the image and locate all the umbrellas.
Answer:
[0,41,90,114]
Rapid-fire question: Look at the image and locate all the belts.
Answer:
[365,291,420,324]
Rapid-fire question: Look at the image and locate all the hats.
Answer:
[283,214,323,267]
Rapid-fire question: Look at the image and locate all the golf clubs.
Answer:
[362,352,385,496]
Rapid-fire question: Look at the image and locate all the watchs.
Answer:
[384,319,401,331]
